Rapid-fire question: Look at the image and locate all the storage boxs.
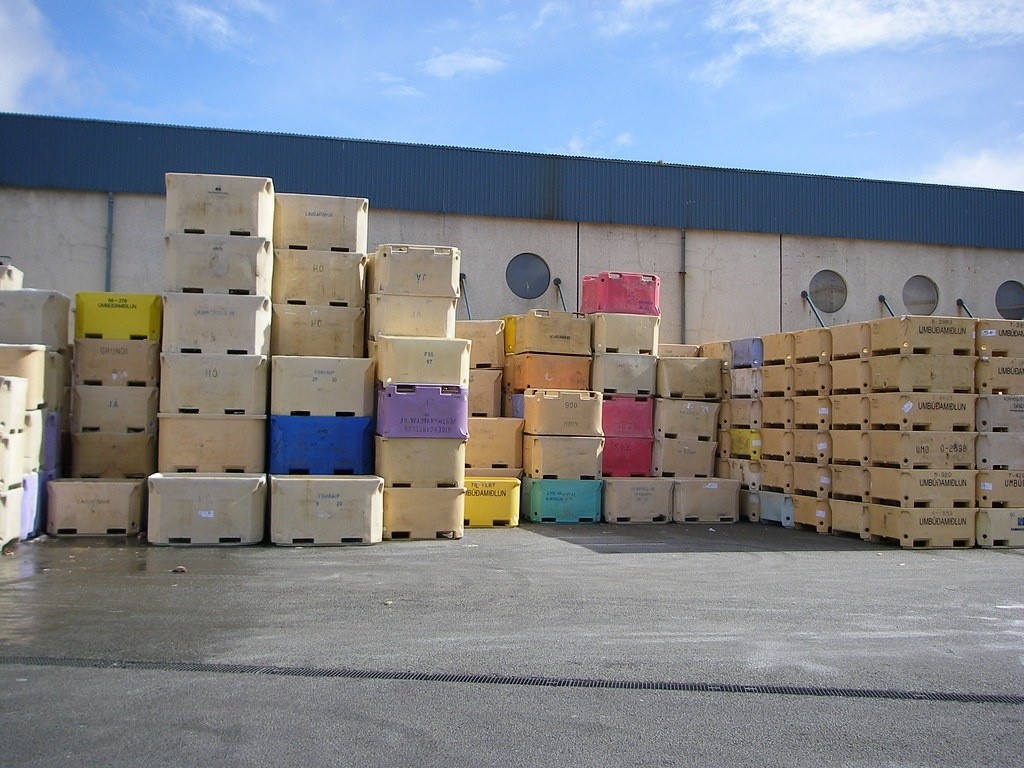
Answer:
[0,169,1024,552]
